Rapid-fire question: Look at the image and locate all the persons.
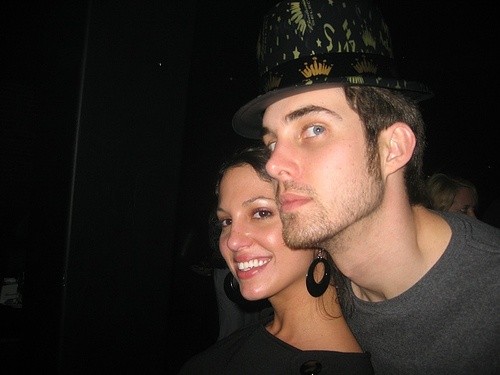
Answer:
[231,0,500,375]
[176,146,376,375]
[426,173,479,220]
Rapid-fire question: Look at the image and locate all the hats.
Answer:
[229,0,434,138]
